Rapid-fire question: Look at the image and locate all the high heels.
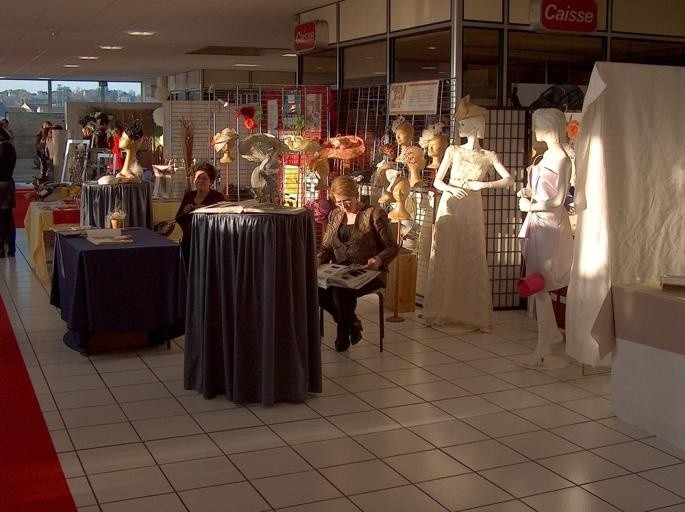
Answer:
[335,320,363,352]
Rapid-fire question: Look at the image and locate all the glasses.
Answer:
[335,201,351,207]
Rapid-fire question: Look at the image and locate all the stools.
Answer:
[321,287,386,354]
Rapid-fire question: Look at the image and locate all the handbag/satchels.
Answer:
[528,84,585,113]
[0,180,16,210]
[517,273,546,298]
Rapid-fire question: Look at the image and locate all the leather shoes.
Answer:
[0,249,15,258]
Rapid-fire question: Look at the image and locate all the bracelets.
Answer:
[489,181,492,189]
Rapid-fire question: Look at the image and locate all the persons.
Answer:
[0,127,17,259]
[96,120,109,146]
[423,98,515,332]
[426,134,448,168]
[115,118,144,184]
[515,108,573,366]
[394,120,415,162]
[387,176,410,223]
[39,119,62,138]
[313,174,400,353]
[175,162,225,280]
[404,145,428,188]
[212,128,237,163]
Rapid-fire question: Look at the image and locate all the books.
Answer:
[188,200,308,216]
[316,262,382,291]
[84,229,134,246]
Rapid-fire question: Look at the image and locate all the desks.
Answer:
[22,200,182,285]
[50,225,189,357]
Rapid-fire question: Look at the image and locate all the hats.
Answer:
[377,116,444,192]
[125,120,144,140]
[211,129,282,162]
[455,96,484,124]
